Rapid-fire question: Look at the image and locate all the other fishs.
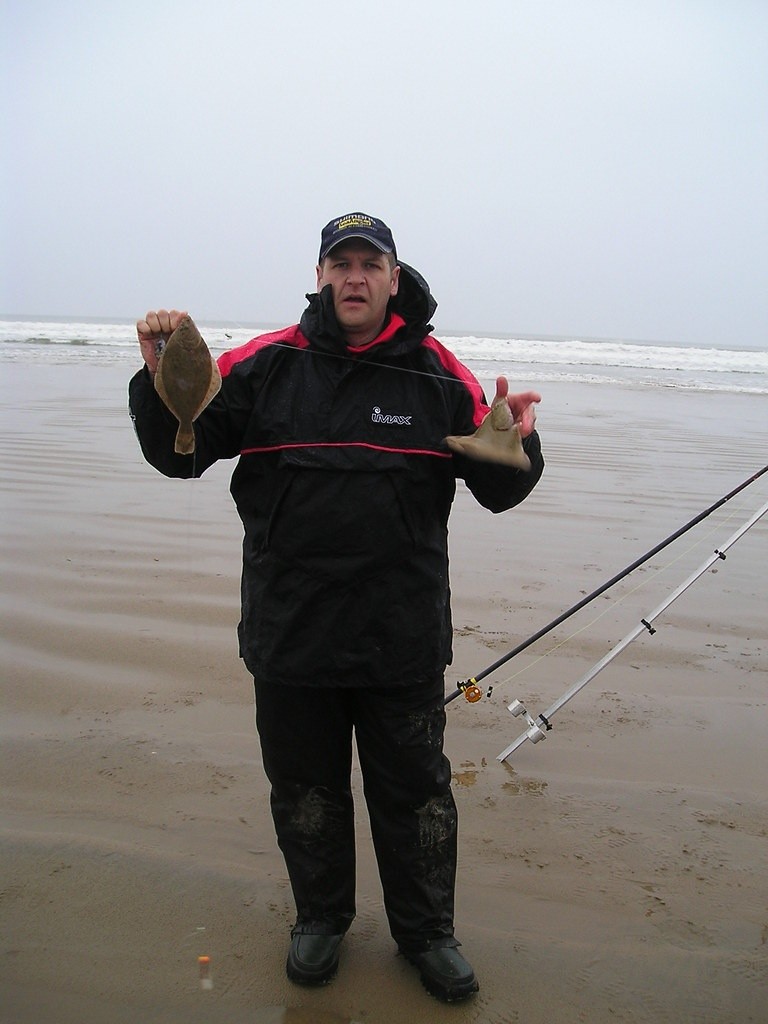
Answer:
[154,316,222,457]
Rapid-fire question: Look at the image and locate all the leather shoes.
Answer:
[398,928,479,1002]
[287,909,355,988]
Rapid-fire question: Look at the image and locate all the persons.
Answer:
[128,209,546,1005]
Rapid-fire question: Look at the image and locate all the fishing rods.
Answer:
[443,464,768,709]
[499,503,768,765]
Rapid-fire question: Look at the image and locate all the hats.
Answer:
[319,213,397,265]
